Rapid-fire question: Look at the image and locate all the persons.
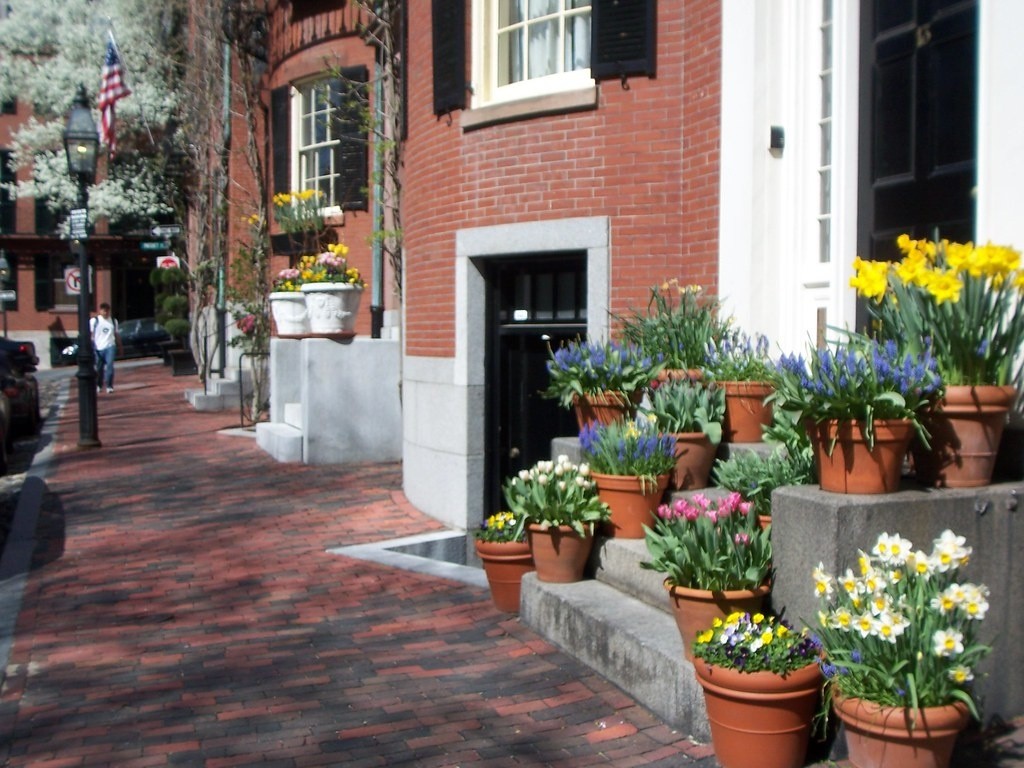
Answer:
[89,303,123,393]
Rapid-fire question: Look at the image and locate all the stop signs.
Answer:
[156,256,181,270]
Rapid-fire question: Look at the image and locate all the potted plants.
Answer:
[150,265,195,379]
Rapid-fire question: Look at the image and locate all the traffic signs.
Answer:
[150,224,182,237]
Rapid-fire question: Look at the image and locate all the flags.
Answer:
[99,39,131,161]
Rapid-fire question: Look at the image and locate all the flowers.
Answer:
[271,244,370,289]
[477,225,1024,745]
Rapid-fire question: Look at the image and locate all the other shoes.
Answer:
[96,385,102,393]
[106,387,114,393]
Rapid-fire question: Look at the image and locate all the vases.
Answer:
[655,431,718,492]
[804,416,913,496]
[908,383,1019,488]
[268,291,312,336]
[687,651,823,768]
[571,390,626,433]
[664,577,772,662]
[474,538,531,613]
[301,283,363,335]
[649,367,703,387]
[755,515,770,532]
[829,682,973,768]
[589,470,670,539]
[521,522,598,585]
[705,380,772,444]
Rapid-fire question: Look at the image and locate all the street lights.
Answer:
[0,250,9,339]
[60,84,104,454]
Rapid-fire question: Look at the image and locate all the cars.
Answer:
[0,337,43,465]
[60,317,167,365]
